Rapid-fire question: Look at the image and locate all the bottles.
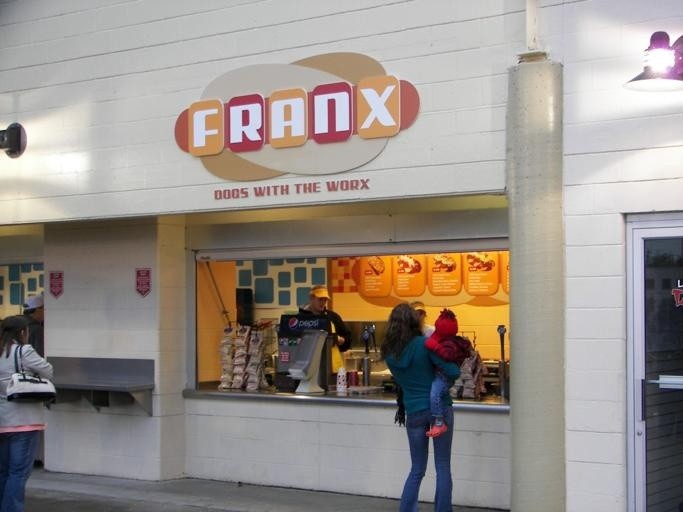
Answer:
[336,365,348,394]
[350,370,359,386]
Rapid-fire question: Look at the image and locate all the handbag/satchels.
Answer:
[6,345,56,401]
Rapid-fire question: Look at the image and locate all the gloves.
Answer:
[425,331,442,351]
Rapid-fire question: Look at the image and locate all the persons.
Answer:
[381,300,473,511]
[0,315,54,512]
[298,286,353,377]
[22,295,44,356]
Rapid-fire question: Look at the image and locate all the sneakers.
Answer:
[426,422,448,437]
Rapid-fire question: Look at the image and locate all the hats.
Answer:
[22,296,44,310]
[310,286,330,300]
[435,308,458,336]
[412,302,426,313]
